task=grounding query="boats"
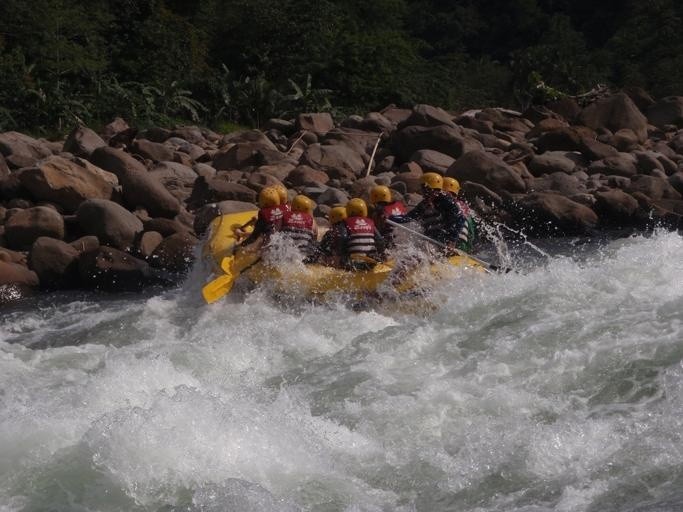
[202,210,487,316]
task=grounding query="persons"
[232,172,476,273]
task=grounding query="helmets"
[258,184,288,208]
[419,172,443,191]
[291,195,312,215]
[346,197,368,217]
[442,177,460,197]
[369,185,392,206]
[329,206,346,225]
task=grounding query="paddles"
[221,235,241,278]
[202,258,262,303]
[384,218,518,275]
